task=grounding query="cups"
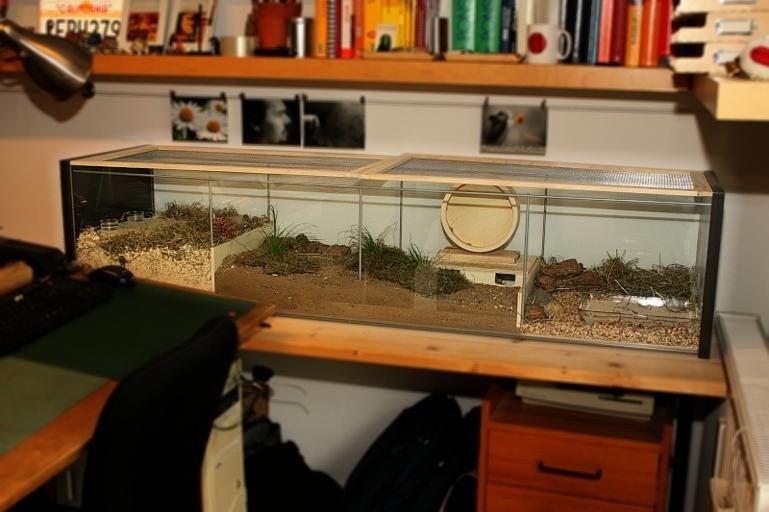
[526,23,571,65]
[288,18,316,57]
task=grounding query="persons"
[169,12,195,49]
[247,99,293,145]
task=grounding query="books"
[313,0,675,70]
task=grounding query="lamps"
[0,19,91,102]
